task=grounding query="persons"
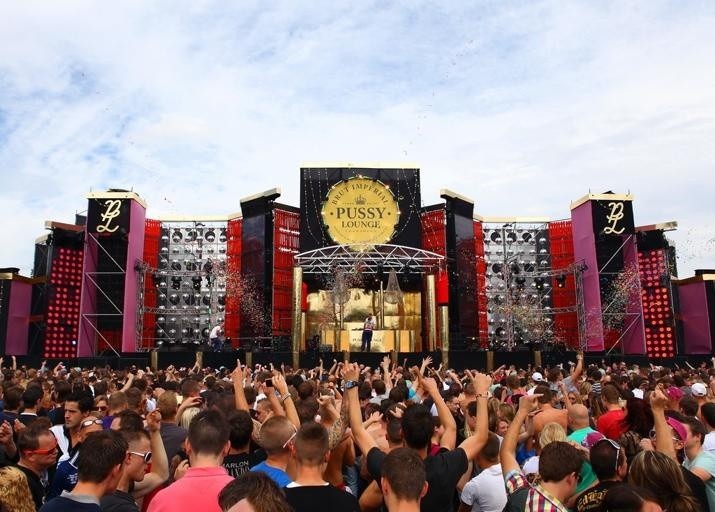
[0,466,34,512]
[126,388,142,415]
[16,427,57,512]
[282,421,362,511]
[443,395,460,422]
[561,360,612,401]
[147,410,237,512]
[629,450,701,512]
[458,435,508,512]
[155,390,189,468]
[500,394,582,512]
[219,471,294,512]
[203,360,345,421]
[96,428,150,511]
[531,386,569,449]
[152,364,204,402]
[492,355,548,403]
[549,355,582,408]
[523,422,567,474]
[361,313,374,353]
[38,429,126,512]
[96,365,152,393]
[17,386,43,428]
[0,363,52,395]
[575,438,627,512]
[180,379,200,428]
[381,448,428,512]
[356,355,475,455]
[621,397,655,464]
[495,417,509,437]
[58,415,103,465]
[0,385,23,423]
[679,396,699,420]
[614,360,715,405]
[48,393,94,461]
[50,364,71,425]
[341,363,492,511]
[93,393,108,417]
[677,417,715,512]
[566,404,607,492]
[103,392,129,428]
[110,410,143,430]
[67,365,96,394]
[597,384,626,440]
[250,415,297,488]
[598,484,662,512]
[700,402,715,455]
[465,401,504,475]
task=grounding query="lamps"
[375,260,385,282]
[403,263,410,284]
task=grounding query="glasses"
[81,419,104,430]
[599,438,621,470]
[283,424,297,449]
[25,443,59,456]
[93,406,109,411]
[649,430,680,442]
[569,397,576,400]
[127,451,153,464]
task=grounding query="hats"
[692,383,707,397]
[532,372,542,381]
[665,417,688,441]
